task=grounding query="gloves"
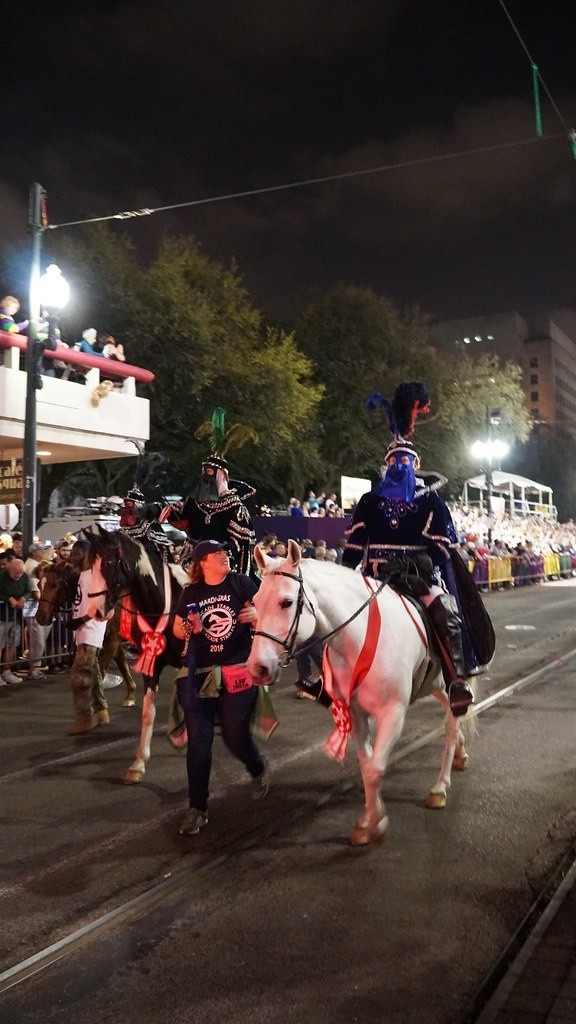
[381,553,413,577]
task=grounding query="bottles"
[434,565,442,586]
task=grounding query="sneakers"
[249,754,270,802]
[0,670,23,686]
[180,807,210,834]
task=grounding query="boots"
[426,594,473,717]
[295,677,332,710]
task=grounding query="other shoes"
[101,672,123,688]
[95,708,110,724]
[44,662,70,674]
[27,669,48,679]
[65,713,98,734]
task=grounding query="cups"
[468,558,475,572]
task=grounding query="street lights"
[475,404,505,551]
[19,184,69,566]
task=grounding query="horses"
[35,523,188,785]
[244,539,478,849]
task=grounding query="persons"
[115,439,474,834]
[0,295,127,393]
[449,507,576,592]
[0,530,110,734]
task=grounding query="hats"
[0,533,11,545]
[194,407,258,475]
[305,538,312,544]
[56,540,69,548]
[124,438,171,504]
[366,380,431,470]
[28,543,43,552]
[191,539,228,562]
[494,539,502,545]
